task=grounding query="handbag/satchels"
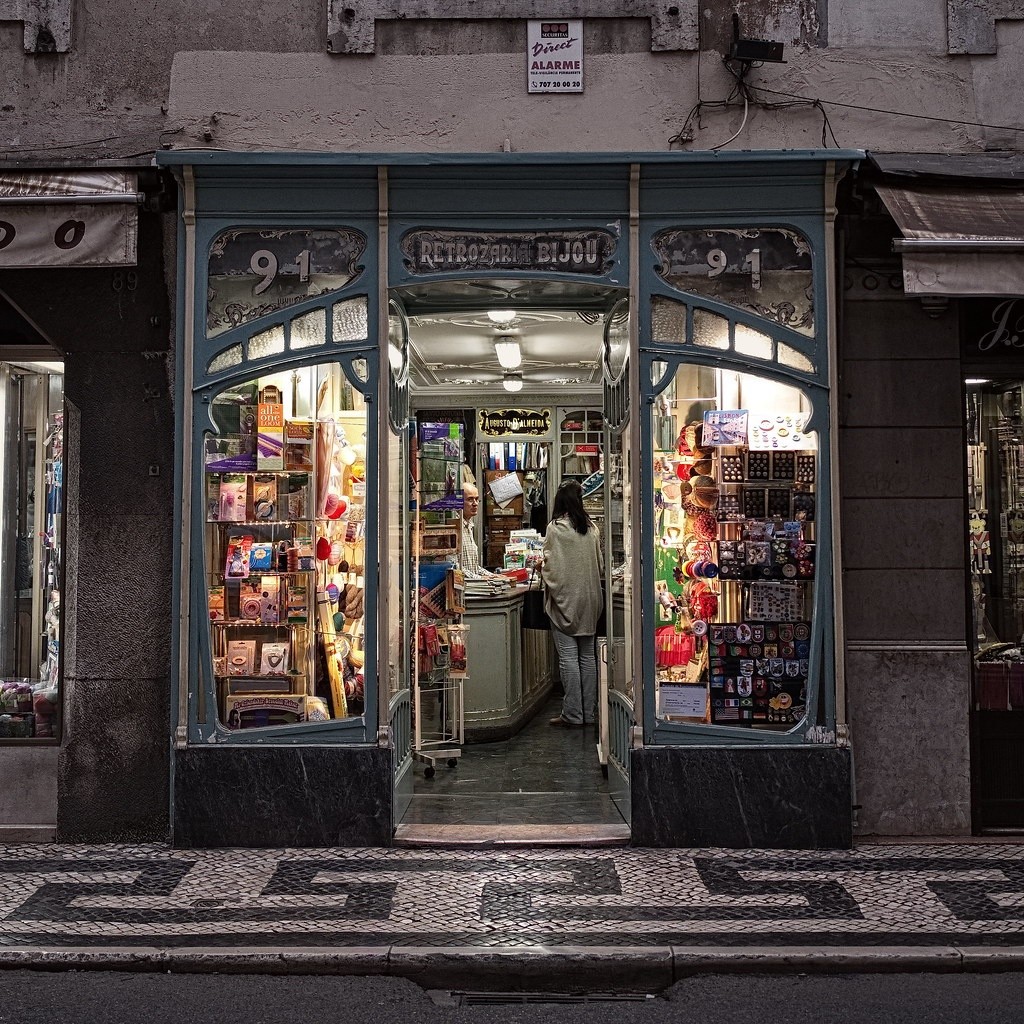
[521,567,557,629]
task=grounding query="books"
[464,577,511,597]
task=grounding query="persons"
[534,478,603,725]
[461,481,507,581]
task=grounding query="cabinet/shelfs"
[458,578,562,730]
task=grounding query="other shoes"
[549,712,578,739]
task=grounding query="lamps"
[502,372,525,392]
[492,335,523,369]
[488,306,519,323]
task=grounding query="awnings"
[869,152,1023,296]
